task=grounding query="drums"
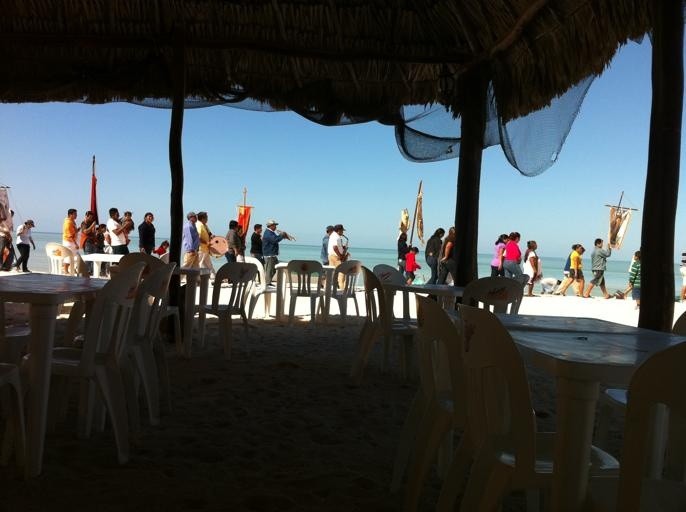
[208,236,229,257]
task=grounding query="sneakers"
[23,270,30,272]
[266,284,275,288]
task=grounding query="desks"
[384,281,463,313]
[445,308,686,512]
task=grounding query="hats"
[187,212,198,219]
[327,226,333,231]
[267,220,278,226]
[28,219,34,227]
[334,225,346,230]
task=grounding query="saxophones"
[342,233,351,266]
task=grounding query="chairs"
[372,263,411,379]
[390,295,473,511]
[461,276,523,315]
[435,303,619,512]
[353,265,418,383]
[589,341,686,512]
[670,312,686,336]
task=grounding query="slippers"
[561,291,565,296]
[584,295,591,298]
[606,295,614,300]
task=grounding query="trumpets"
[275,225,293,241]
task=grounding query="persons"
[680,253,686,302]
[225,221,295,288]
[629,251,642,309]
[320,226,335,290]
[196,212,219,286]
[404,247,421,286]
[491,231,543,296]
[0,210,14,272]
[424,228,444,286]
[328,224,345,291]
[398,233,407,275]
[540,277,561,296]
[13,220,35,273]
[181,212,200,285]
[615,247,642,299]
[431,226,456,302]
[155,240,169,255]
[139,212,156,256]
[586,239,612,297]
[62,208,134,274]
